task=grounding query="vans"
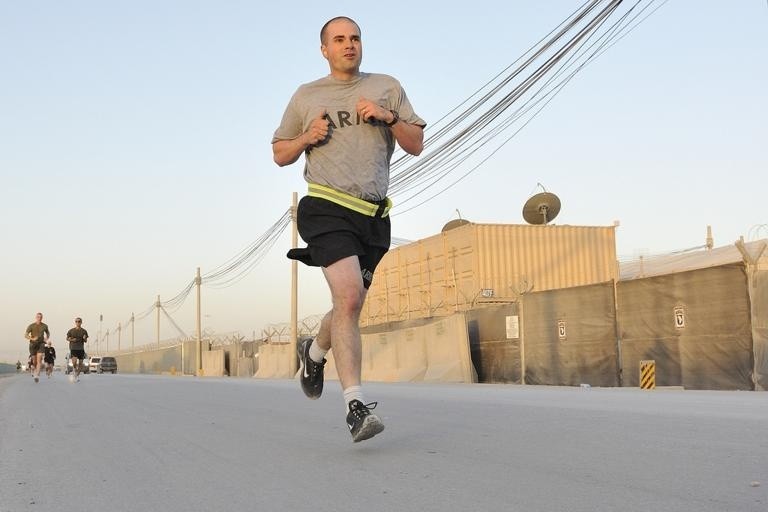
[64,351,91,374]
[88,356,102,373]
[96,356,119,375]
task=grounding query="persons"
[271,16,427,442]
[16,312,88,384]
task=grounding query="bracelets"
[384,109,399,127]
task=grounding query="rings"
[362,109,365,113]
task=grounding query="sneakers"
[346,398,385,443]
[295,336,327,400]
[28,370,81,384]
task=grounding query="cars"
[54,364,62,371]
